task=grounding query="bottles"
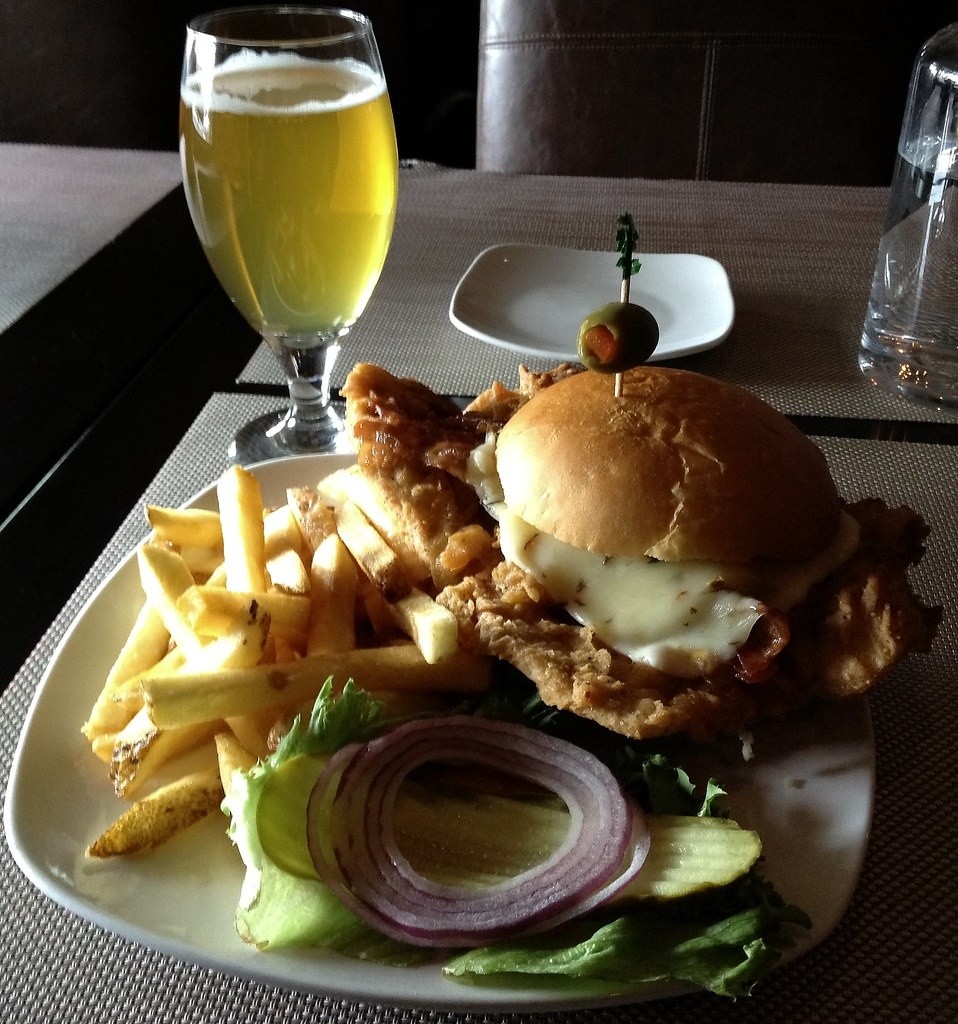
[858,21,958,406]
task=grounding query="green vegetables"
[247,675,812,1001]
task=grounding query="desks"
[1,141,958,1024]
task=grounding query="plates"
[6,453,878,1008]
[447,241,735,366]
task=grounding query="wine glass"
[180,7,399,474]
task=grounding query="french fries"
[86,465,464,861]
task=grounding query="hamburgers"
[484,371,839,682]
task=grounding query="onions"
[303,715,654,945]
